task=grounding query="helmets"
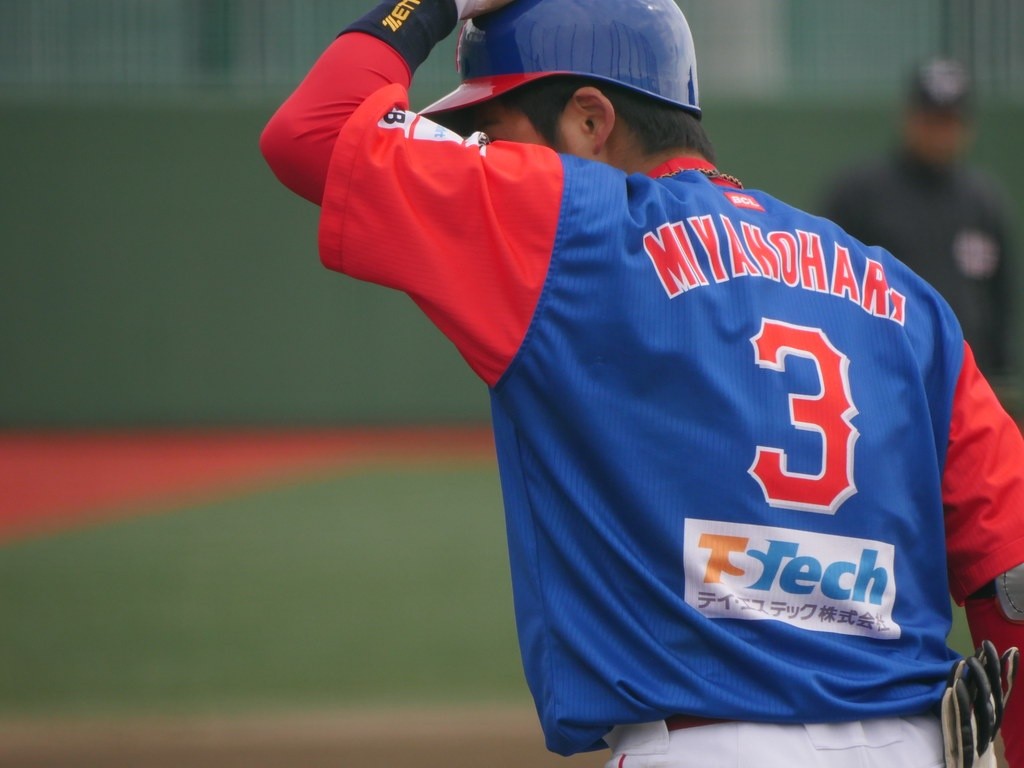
[413,1,705,115]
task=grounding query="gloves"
[941,638,1021,768]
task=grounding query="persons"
[253,0,1024,767]
[814,53,1018,424]
